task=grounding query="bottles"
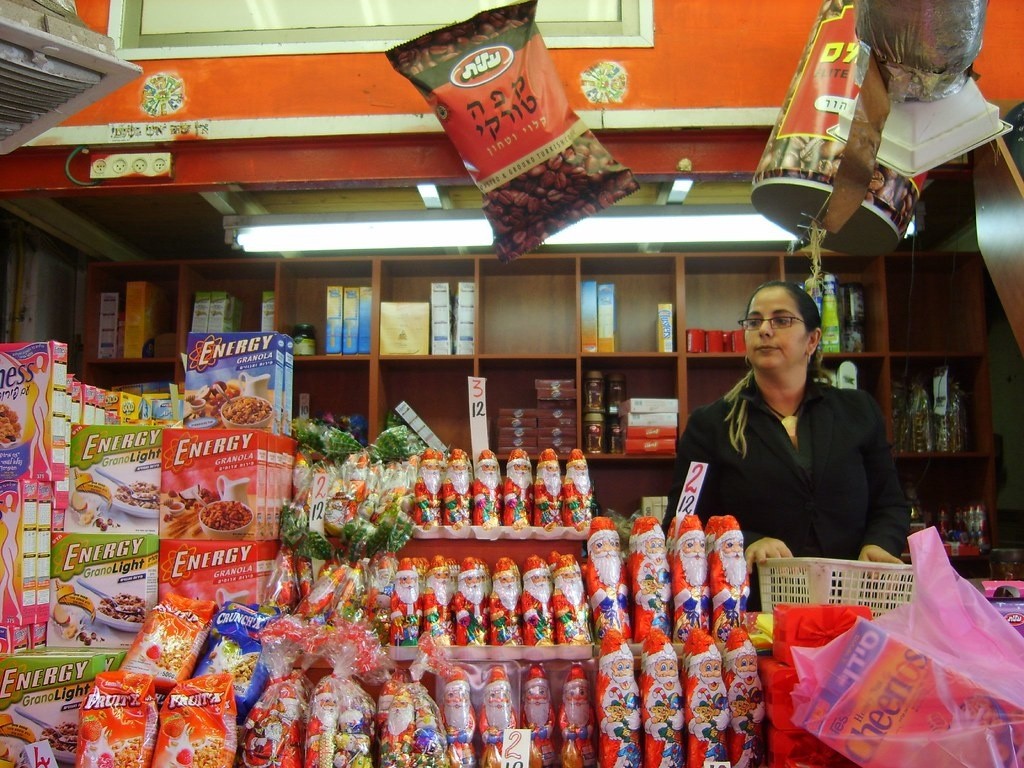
[805,274,841,353]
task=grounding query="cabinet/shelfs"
[82,253,999,581]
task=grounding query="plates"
[24,726,76,763]
[86,594,144,632]
[184,401,191,418]
[101,481,160,518]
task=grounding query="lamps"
[223,204,811,252]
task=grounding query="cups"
[189,399,206,413]
[166,502,185,516]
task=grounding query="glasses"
[737,317,809,331]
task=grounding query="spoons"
[94,468,157,500]
[77,578,141,613]
[14,707,78,744]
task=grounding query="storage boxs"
[655,303,674,352]
[191,291,246,333]
[393,400,448,453]
[300,393,311,420]
[1,341,184,768]
[580,280,619,352]
[494,378,578,455]
[641,496,668,522]
[326,286,372,355]
[98,281,171,359]
[261,291,275,332]
[813,361,857,390]
[159,331,299,621]
[619,398,680,454]
[430,282,475,356]
[380,302,429,355]
[686,329,747,354]
[805,617,1024,768]
[944,543,980,556]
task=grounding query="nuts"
[478,131,638,261]
[752,136,916,239]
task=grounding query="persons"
[660,281,909,575]
[243,447,765,768]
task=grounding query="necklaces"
[763,397,804,437]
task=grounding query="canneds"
[579,368,626,455]
[687,328,746,353]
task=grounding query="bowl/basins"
[198,499,254,540]
[221,395,273,429]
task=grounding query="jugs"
[216,475,250,501]
[238,372,271,400]
[215,588,249,610]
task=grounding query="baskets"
[755,557,915,620]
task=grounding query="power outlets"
[89,153,174,180]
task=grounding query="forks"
[186,395,195,402]
[159,500,173,506]
